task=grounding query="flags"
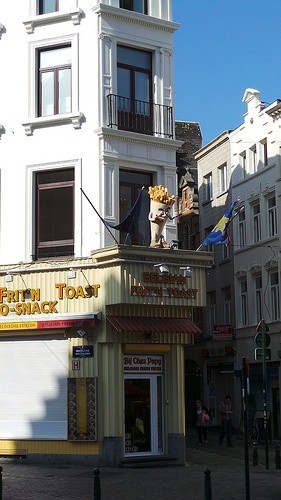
[202,201,238,247]
[104,191,142,232]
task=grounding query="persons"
[192,400,211,446]
[219,394,234,448]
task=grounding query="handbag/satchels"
[203,413,210,422]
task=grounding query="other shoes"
[219,438,222,445]
[227,444,233,448]
